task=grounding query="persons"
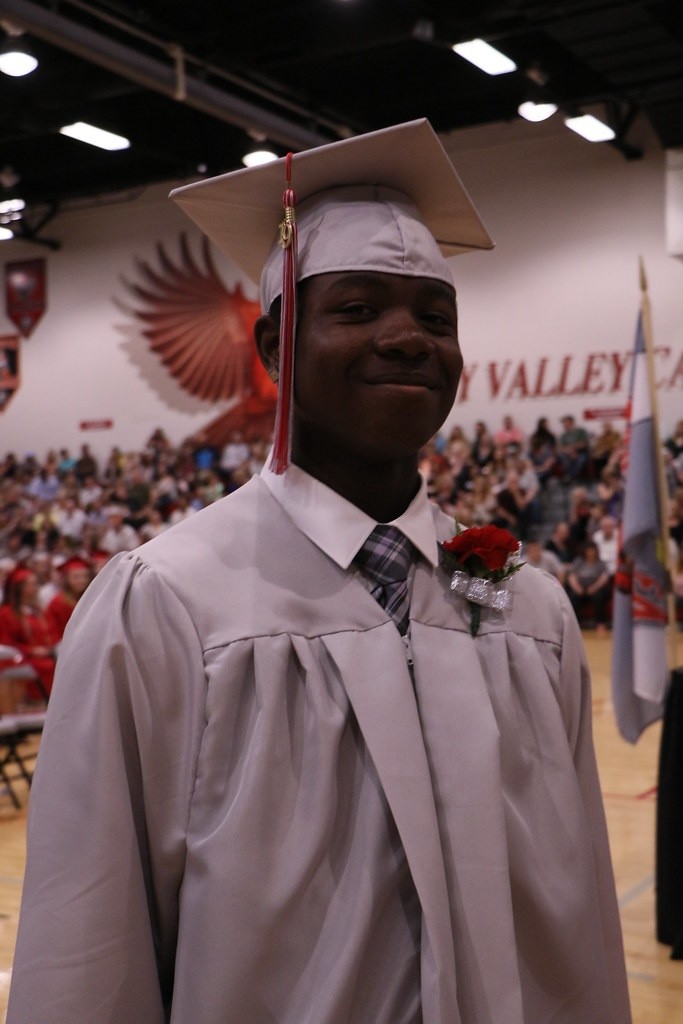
[0,115,650,1024]
[649,533,683,970]
[0,398,683,711]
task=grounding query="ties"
[358,526,416,638]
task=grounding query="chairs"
[0,646,49,809]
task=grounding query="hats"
[4,549,111,584]
[167,118,496,475]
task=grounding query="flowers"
[435,515,529,639]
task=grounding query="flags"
[0,332,22,414]
[605,299,668,748]
[2,255,50,341]
